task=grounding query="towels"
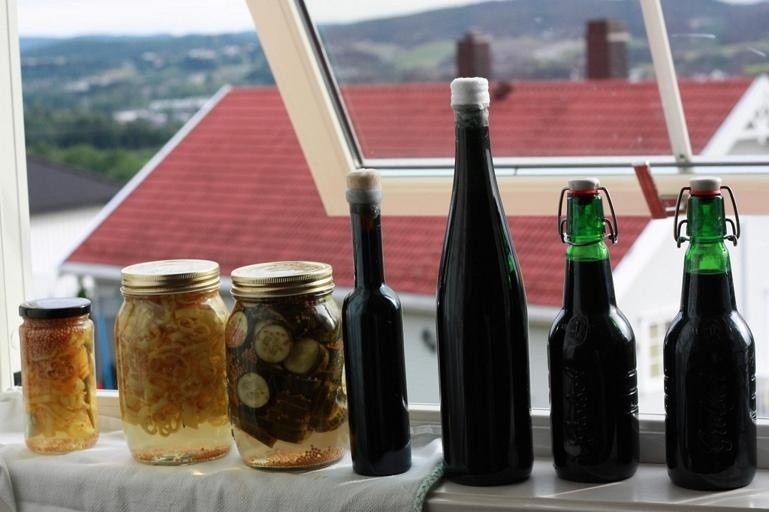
[1,411,445,511]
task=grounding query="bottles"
[543,176,641,485]
[657,176,761,491]
[433,76,537,489]
[336,168,414,478]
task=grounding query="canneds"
[18,295,98,453]
[224,256,347,467]
[113,258,228,465]
